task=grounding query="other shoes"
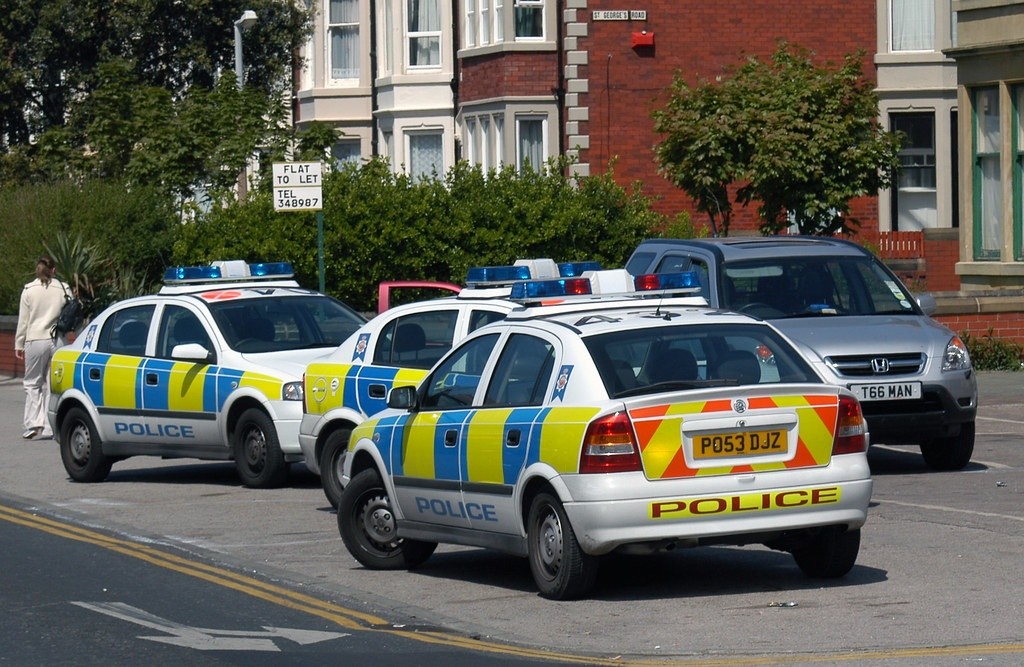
[37,434,53,440]
[23,426,38,439]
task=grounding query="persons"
[14,257,75,438]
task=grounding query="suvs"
[624,235,980,469]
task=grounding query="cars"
[296,257,705,506]
[44,259,392,490]
[337,268,875,601]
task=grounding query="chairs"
[118,321,149,351]
[598,360,637,393]
[237,318,275,341]
[712,350,761,384]
[393,323,426,362]
[173,318,208,346]
[647,348,698,384]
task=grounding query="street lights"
[233,11,258,206]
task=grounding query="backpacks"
[50,282,84,339]
